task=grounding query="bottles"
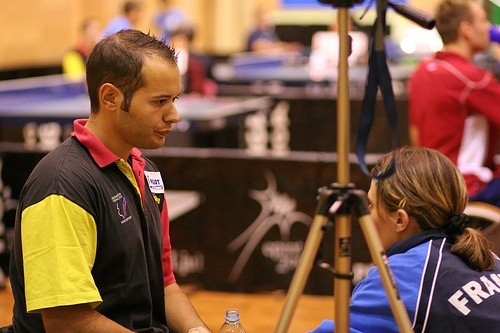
[218,310,246,333]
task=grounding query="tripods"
[274,0,416,333]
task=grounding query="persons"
[149,1,188,49]
[98,0,143,43]
[307,147,500,333]
[61,17,102,79]
[10,29,213,333]
[407,0,500,239]
